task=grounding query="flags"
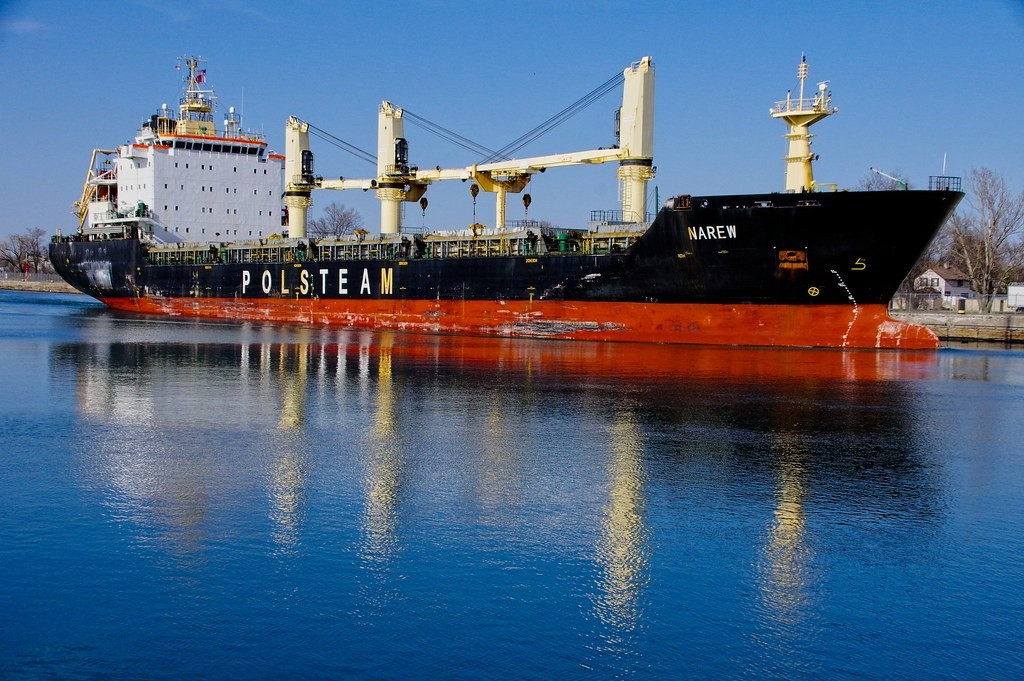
[196,69,206,75]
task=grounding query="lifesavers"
[290,253,295,260]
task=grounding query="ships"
[43,57,967,347]
